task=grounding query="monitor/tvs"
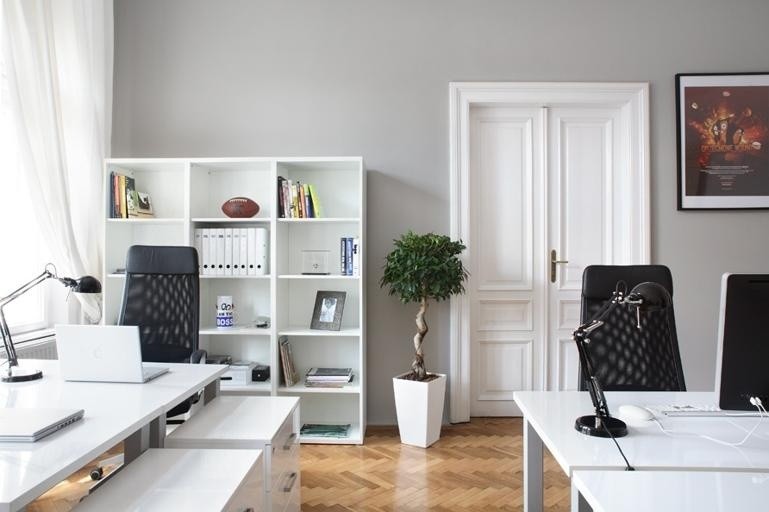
[713,272,769,411]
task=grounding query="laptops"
[0,407,84,443]
[53,323,171,384]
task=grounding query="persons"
[320,298,336,322]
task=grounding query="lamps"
[0,263,102,381]
[573,280,672,437]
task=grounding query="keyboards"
[655,399,769,418]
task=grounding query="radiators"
[0,334,58,360]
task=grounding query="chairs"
[577,264,687,391]
[80,245,232,501]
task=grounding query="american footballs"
[221,198,259,218]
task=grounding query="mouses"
[619,404,654,422]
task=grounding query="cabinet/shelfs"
[68,448,266,512]
[103,157,368,445]
[164,395,302,511]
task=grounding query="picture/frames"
[310,291,345,331]
[675,72,769,211]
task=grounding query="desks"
[0,405,164,511]
[572,469,769,512]
[512,391,769,512]
[0,357,230,448]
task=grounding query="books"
[277,175,320,218]
[277,335,356,388]
[340,236,360,277]
[110,171,154,219]
[300,423,352,439]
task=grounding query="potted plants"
[378,226,472,451]
